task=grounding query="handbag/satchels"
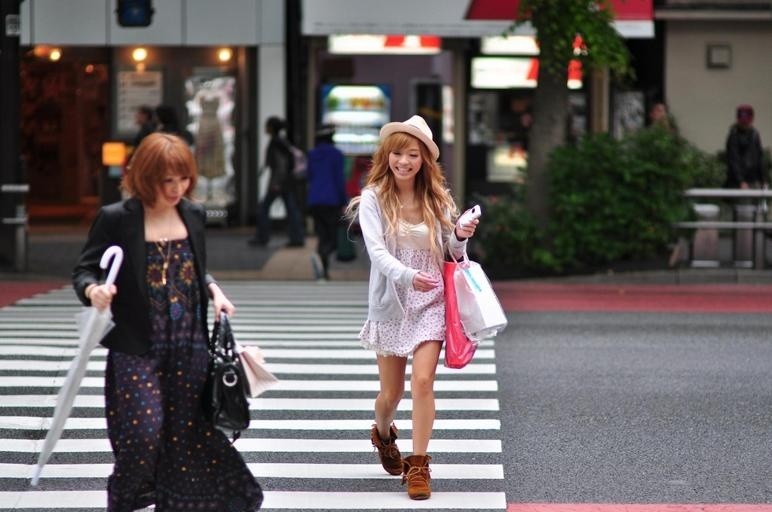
[208,311,250,431]
[336,224,357,263]
[454,261,509,345]
[237,344,278,399]
[443,261,478,368]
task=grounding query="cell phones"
[459,204,482,229]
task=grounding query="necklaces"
[149,207,173,286]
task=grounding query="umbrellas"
[29,245,124,487]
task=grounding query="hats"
[380,115,440,161]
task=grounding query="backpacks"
[285,142,310,184]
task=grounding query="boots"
[371,423,403,476]
[400,455,432,499]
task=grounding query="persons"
[247,92,441,281]
[647,102,671,133]
[721,104,765,270]
[509,95,533,148]
[115,106,157,155]
[154,105,197,147]
[70,132,264,512]
[343,113,479,500]
[197,76,225,177]
